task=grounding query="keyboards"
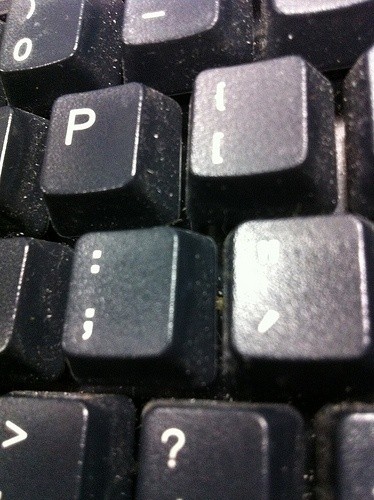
[1,1,374,500]
